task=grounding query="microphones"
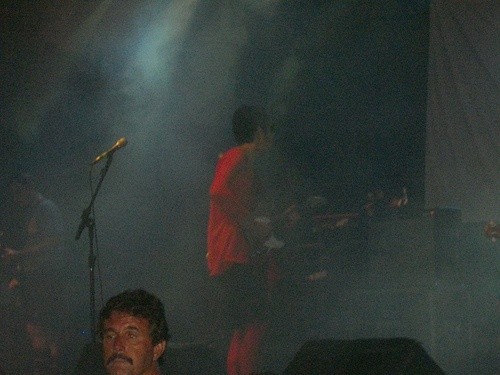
[88,137,127,165]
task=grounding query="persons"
[207,103,301,374]
[1,171,68,375]
[98,288,170,375]
